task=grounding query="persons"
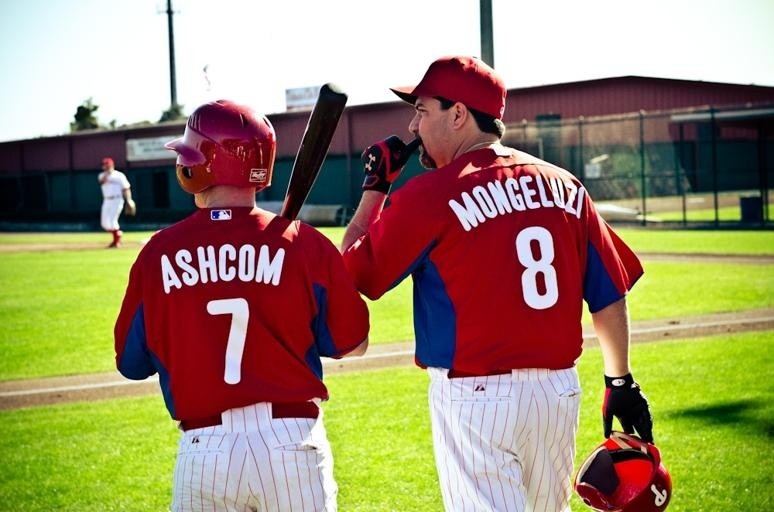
[114,100,371,512]
[97,157,135,247]
[340,55,654,512]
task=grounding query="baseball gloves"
[125,200,136,217]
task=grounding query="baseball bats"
[280,84,348,220]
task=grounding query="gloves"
[602,375,652,444]
[360,136,421,196]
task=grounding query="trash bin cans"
[740,196,762,223]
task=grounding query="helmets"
[575,430,671,512]
[165,99,277,194]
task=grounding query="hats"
[390,56,508,120]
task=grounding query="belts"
[182,402,319,432]
